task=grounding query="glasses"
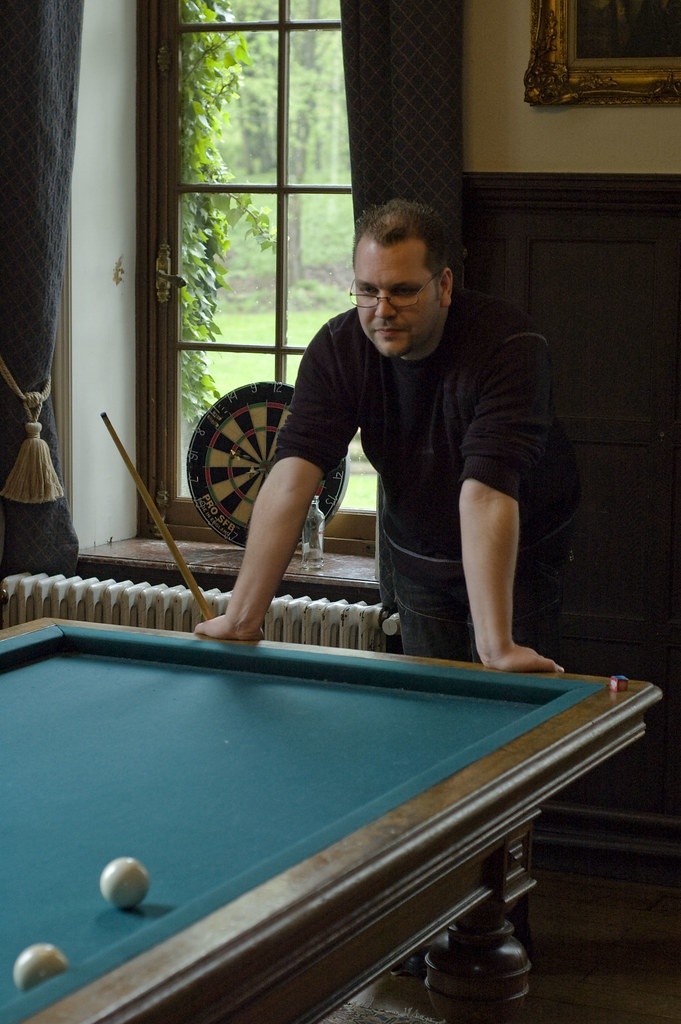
[350,272,439,308]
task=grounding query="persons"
[194,201,580,980]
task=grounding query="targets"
[186,381,352,549]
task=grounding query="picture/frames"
[523,0,681,107]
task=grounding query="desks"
[0,616,662,1024]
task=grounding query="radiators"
[0,572,402,651]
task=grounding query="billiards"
[99,856,150,912]
[12,941,68,992]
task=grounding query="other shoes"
[406,952,427,980]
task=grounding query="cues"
[100,412,215,621]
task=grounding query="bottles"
[301,496,324,569]
